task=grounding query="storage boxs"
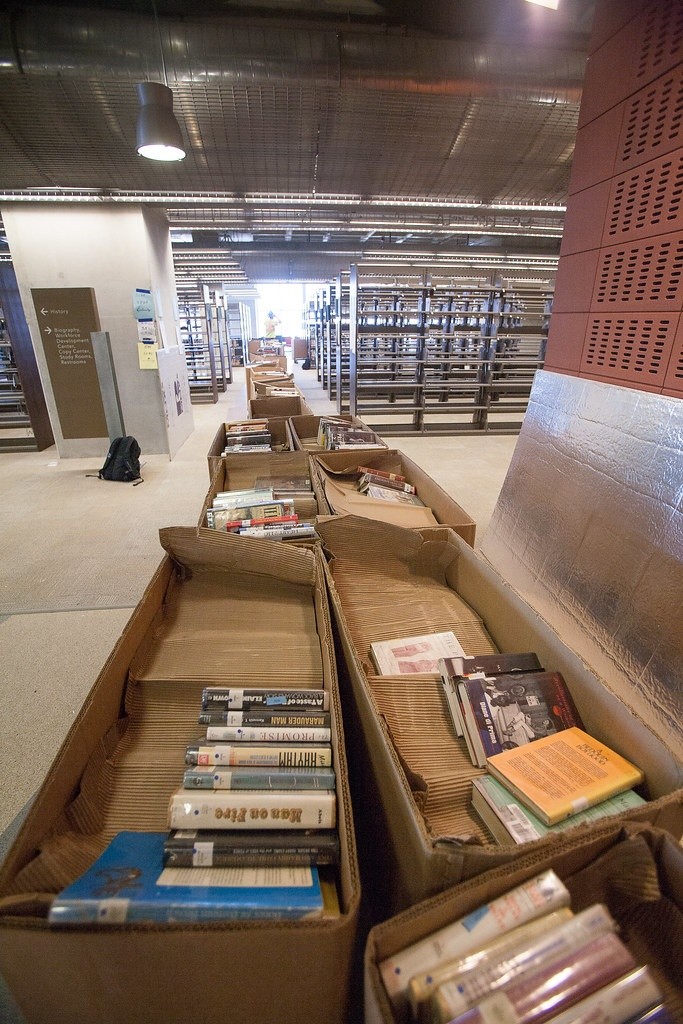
[1,353,683,1024]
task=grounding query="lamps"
[136,81,186,162]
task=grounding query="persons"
[264,310,281,338]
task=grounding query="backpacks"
[99,436,141,482]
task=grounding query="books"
[221,420,271,456]
[317,416,386,450]
[380,869,672,1024]
[471,726,648,847]
[370,631,466,676]
[438,652,587,767]
[50,686,337,922]
[261,371,285,375]
[208,475,317,541]
[271,388,298,397]
[347,466,425,507]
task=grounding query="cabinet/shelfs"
[173,260,559,436]
